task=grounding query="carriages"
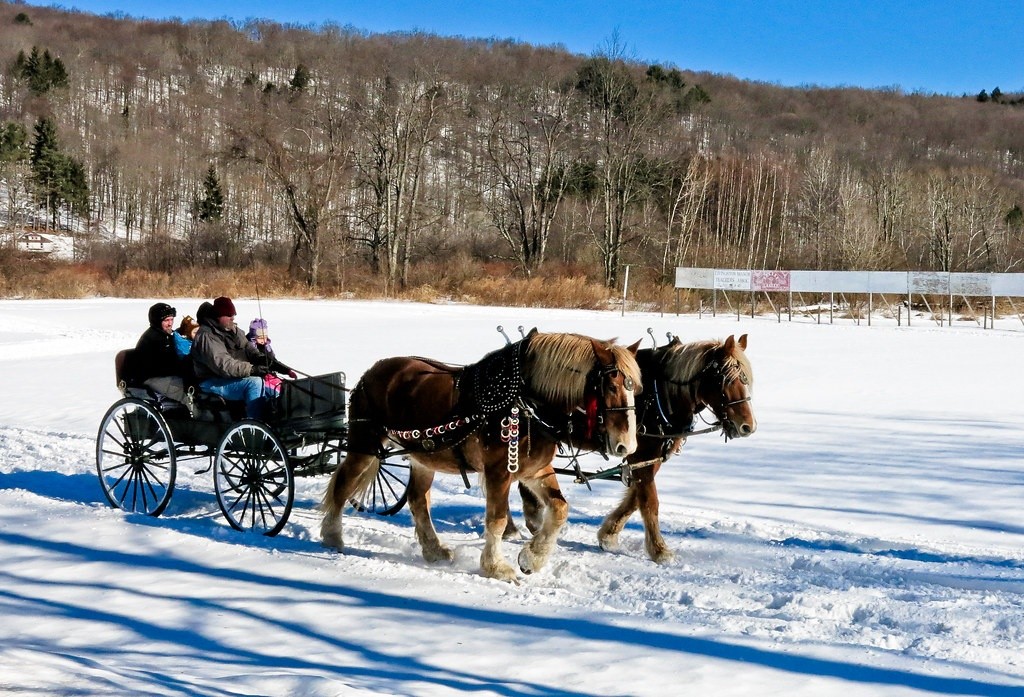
[95,325,758,582]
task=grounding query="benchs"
[186,383,286,412]
[114,350,185,411]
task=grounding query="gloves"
[286,369,298,380]
[254,365,269,377]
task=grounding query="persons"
[134,303,202,419]
[245,318,282,392]
[191,297,297,420]
[179,316,200,341]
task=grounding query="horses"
[519,332,756,569]
[319,330,644,583]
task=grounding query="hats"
[180,315,200,342]
[149,303,176,331]
[214,298,237,318]
[249,319,272,354]
